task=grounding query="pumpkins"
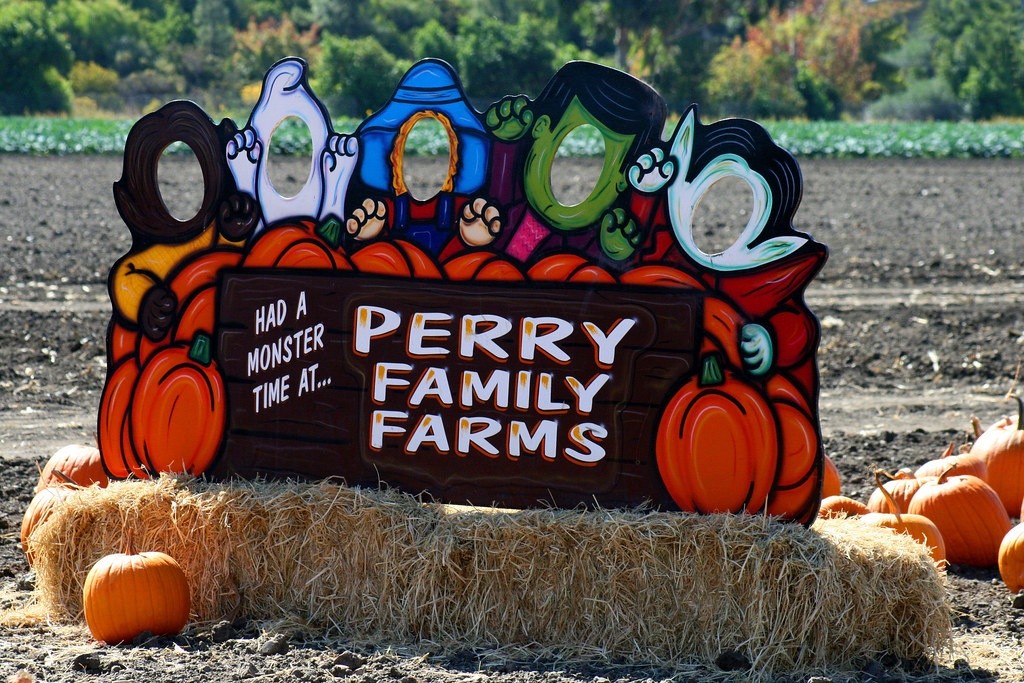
[20,431,108,565]
[83,526,190,644]
[816,394,1024,594]
